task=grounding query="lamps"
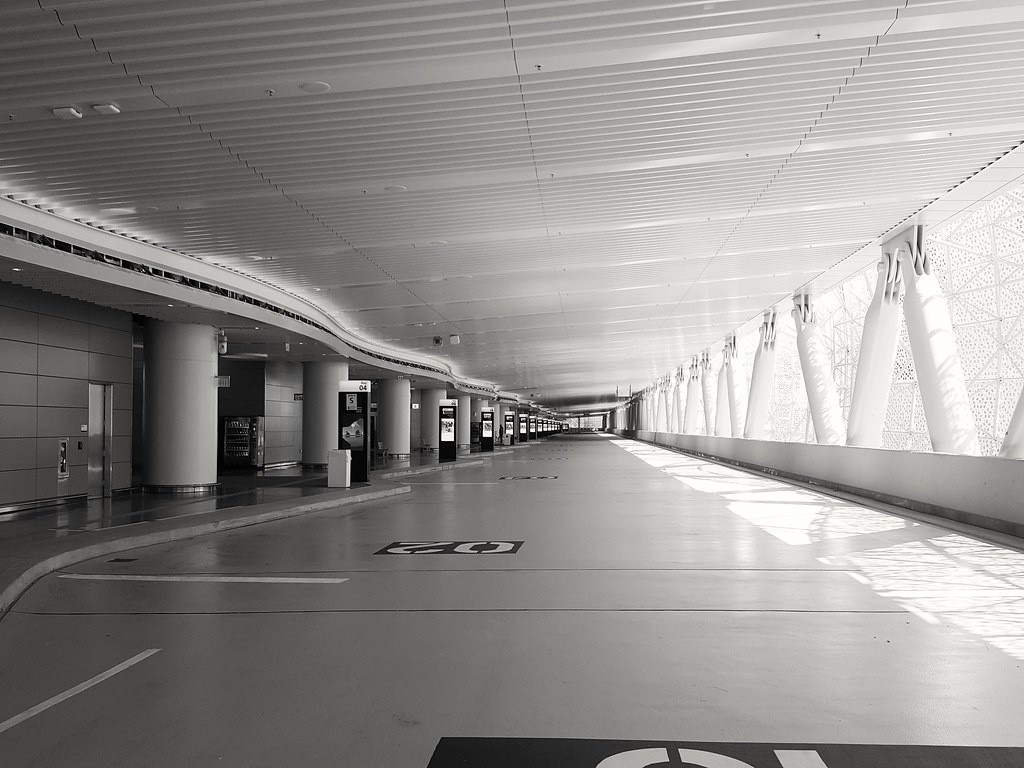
[215,330,228,354]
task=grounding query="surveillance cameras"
[433,337,443,346]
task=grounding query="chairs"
[376,440,391,458]
[421,439,432,452]
